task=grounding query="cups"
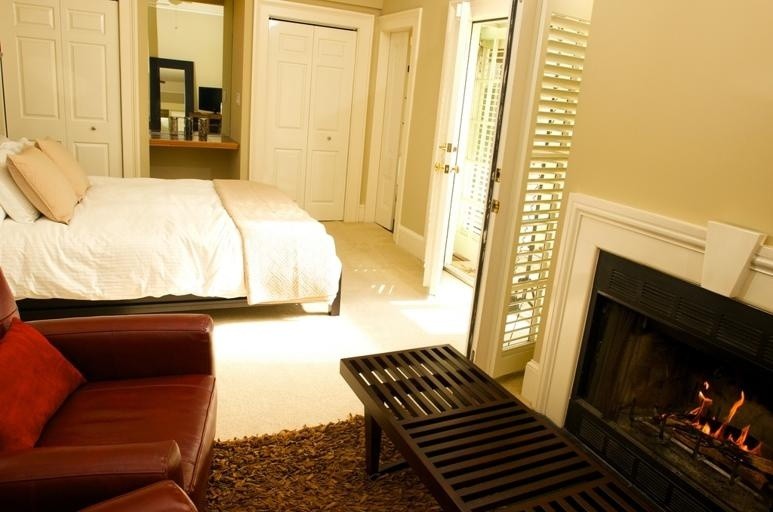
[184,117,193,141]
[168,117,177,137]
[198,117,208,141]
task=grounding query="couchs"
[75,479,198,512]
[0,264,218,512]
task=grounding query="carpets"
[210,403,445,511]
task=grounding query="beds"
[0,136,343,317]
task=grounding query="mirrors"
[148,0,224,138]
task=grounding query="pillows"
[7,143,84,226]
[34,139,91,196]
[0,143,42,226]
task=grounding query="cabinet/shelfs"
[267,17,357,222]
[0,0,124,179]
